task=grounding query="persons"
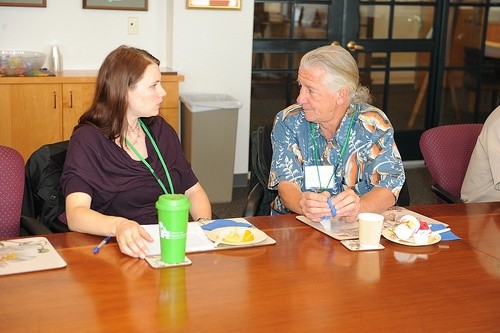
[60,45,212,259]
[268,45,407,223]
[460,101,500,202]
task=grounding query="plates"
[208,226,266,245]
[382,226,441,247]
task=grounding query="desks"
[0,199,500,333]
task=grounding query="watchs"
[197,217,210,221]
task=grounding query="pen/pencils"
[327,197,336,216]
[93,237,110,253]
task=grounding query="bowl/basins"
[0,50,46,76]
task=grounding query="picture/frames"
[187,0,241,10]
[82,0,149,12]
[0,0,47,7]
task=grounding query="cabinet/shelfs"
[0,70,185,165]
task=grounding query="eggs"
[394,214,420,240]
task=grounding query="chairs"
[0,123,485,240]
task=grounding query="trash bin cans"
[180,93,240,204]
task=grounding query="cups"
[155,193,191,264]
[394,215,421,241]
[49,45,63,73]
[358,213,384,249]
